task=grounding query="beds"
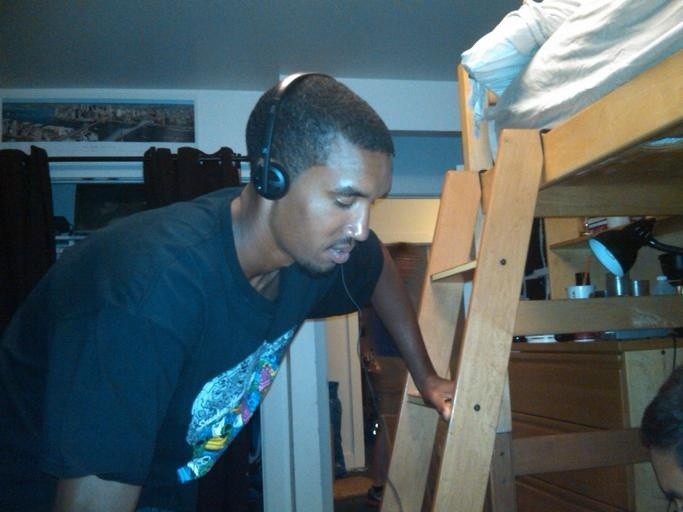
[458,1,683,213]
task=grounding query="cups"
[565,284,596,343]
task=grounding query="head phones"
[253,71,333,200]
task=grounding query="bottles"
[648,275,676,337]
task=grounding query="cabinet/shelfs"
[508,336,683,512]
[543,216,683,299]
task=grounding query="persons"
[639,363,683,512]
[0,73,456,512]
